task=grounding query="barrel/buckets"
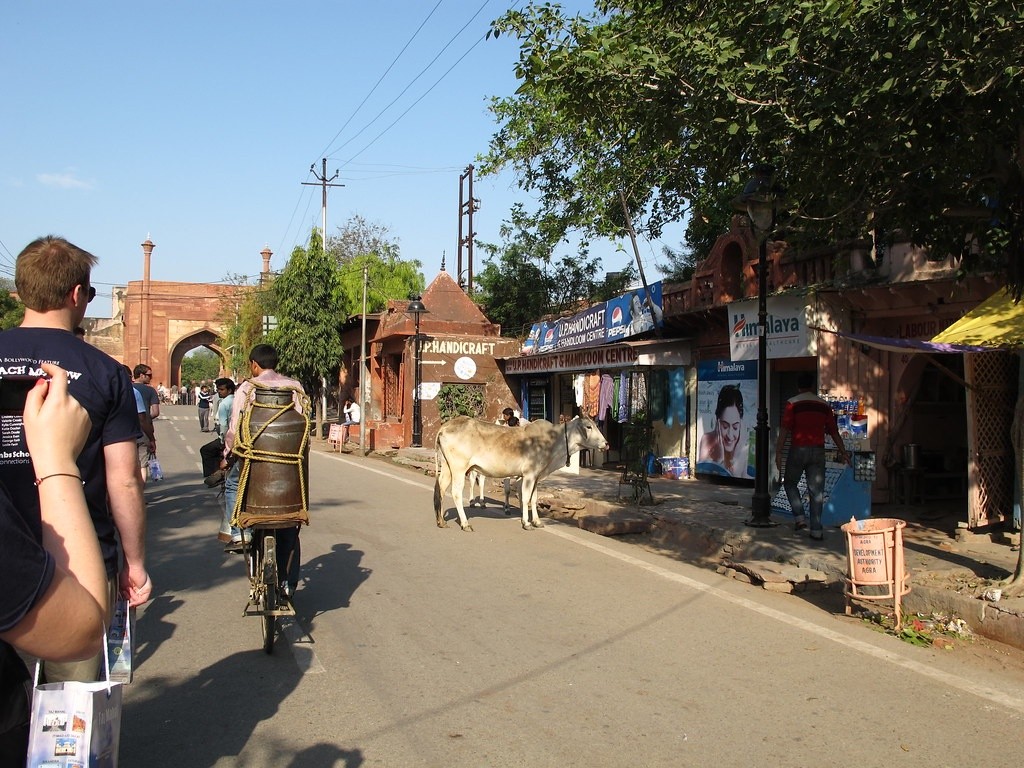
[238,392,309,523]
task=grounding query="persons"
[158,382,165,403]
[342,398,360,440]
[0,363,110,768]
[699,385,749,477]
[776,379,851,539]
[172,379,223,435]
[1,236,160,682]
[624,294,662,337]
[200,378,235,488]
[224,345,306,609]
[503,409,530,426]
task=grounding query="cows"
[434,405,610,532]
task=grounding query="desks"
[893,466,966,506]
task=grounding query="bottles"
[817,393,866,416]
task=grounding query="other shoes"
[204,470,227,489]
[810,534,823,540]
[795,520,808,530]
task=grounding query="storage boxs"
[660,456,689,480]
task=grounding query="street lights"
[402,295,431,448]
[738,163,782,528]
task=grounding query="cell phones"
[0,375,51,417]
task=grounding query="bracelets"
[34,473,85,488]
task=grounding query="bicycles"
[222,458,298,654]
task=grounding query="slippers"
[224,540,248,552]
[280,595,294,607]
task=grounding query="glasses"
[144,373,152,377]
[84,286,96,302]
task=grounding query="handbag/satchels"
[148,452,163,482]
[26,626,123,768]
[100,591,135,684]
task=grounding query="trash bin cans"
[840,518,914,633]
[328,423,352,454]
